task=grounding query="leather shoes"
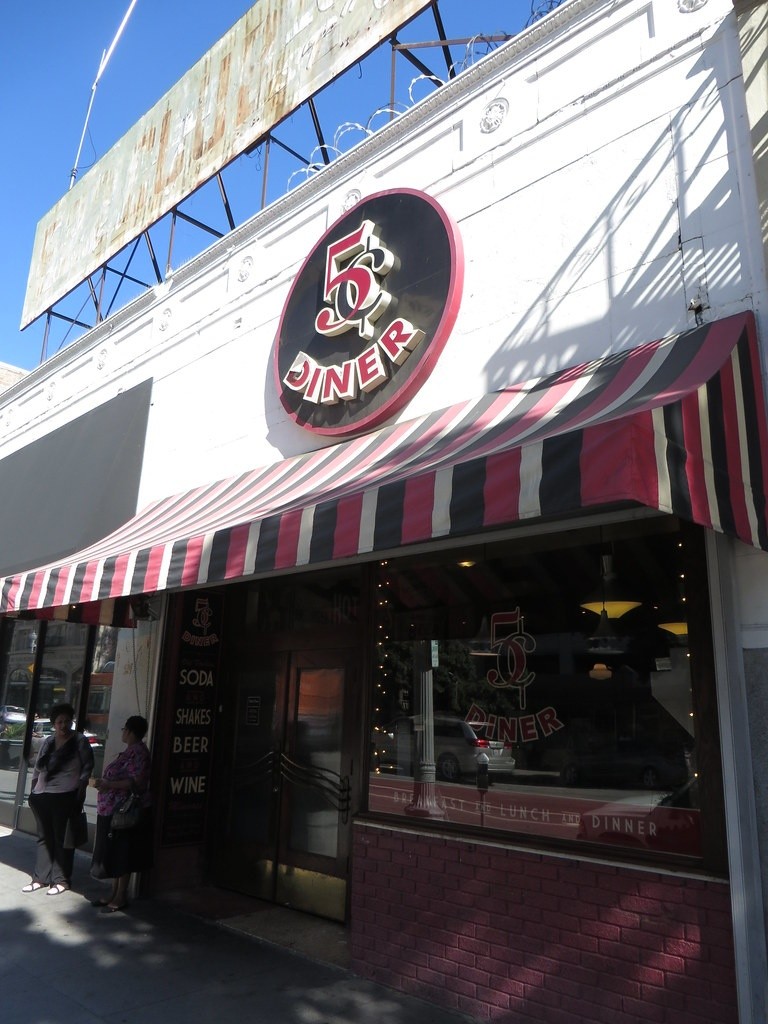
[91,895,118,907]
[101,900,128,913]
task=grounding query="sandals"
[46,884,66,895]
[22,881,49,892]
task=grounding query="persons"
[22,704,94,895]
[92,715,150,914]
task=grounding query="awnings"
[0,311,767,626]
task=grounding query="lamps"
[454,523,689,677]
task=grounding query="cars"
[0,704,26,723]
[0,718,103,764]
[371,715,515,783]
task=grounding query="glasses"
[35,716,39,718]
[121,728,127,731]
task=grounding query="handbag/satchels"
[110,777,141,830]
[63,798,89,849]
